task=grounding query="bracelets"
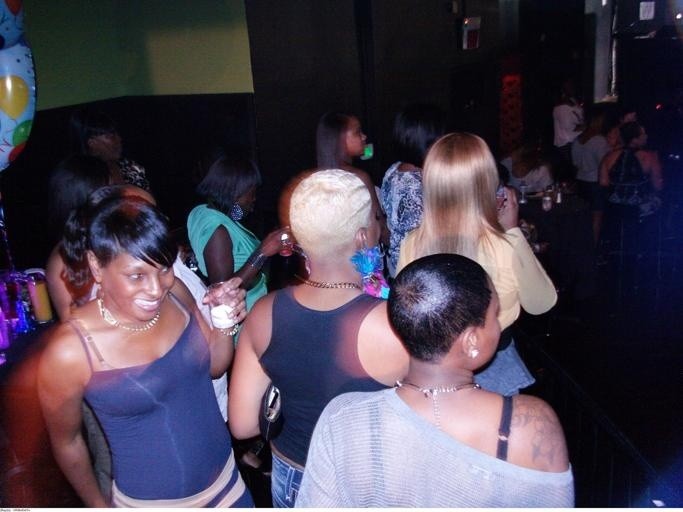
[219,323,240,336]
[247,249,267,272]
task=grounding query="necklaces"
[96,298,161,331]
[302,279,364,291]
[397,377,481,436]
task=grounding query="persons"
[381,104,450,279]
[185,153,297,471]
[1,185,230,507]
[548,75,584,167]
[228,167,410,508]
[599,122,665,219]
[396,128,558,397]
[36,196,254,507]
[316,110,391,249]
[294,254,575,509]
[498,136,554,195]
[52,122,151,244]
[483,133,510,185]
[608,108,638,152]
[571,111,614,246]
[1,131,231,211]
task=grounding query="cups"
[206,282,238,336]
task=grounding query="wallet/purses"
[259,383,282,441]
